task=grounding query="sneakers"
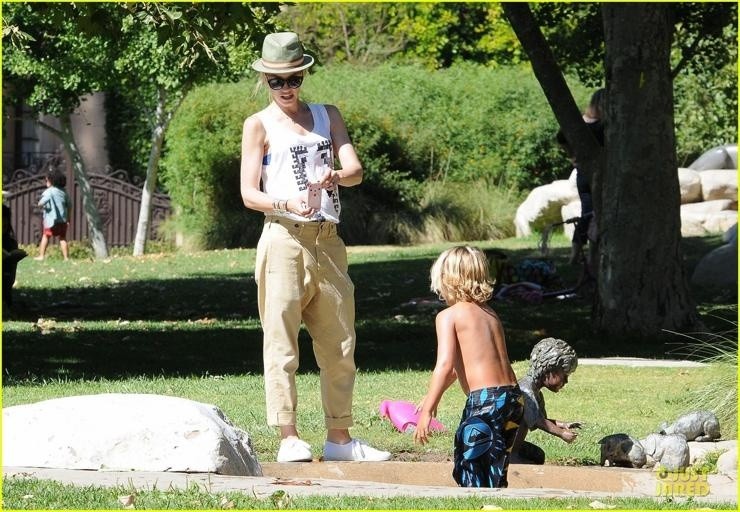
[277,435,313,462]
[323,438,393,462]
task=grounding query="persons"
[567,88,607,264]
[239,33,397,464]
[510,335,583,465]
[412,243,525,484]
[33,169,72,262]
[2,202,28,305]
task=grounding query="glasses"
[265,71,304,90]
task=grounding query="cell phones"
[306,180,322,210]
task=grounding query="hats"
[47,169,66,188]
[251,31,315,75]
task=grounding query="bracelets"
[272,199,289,212]
